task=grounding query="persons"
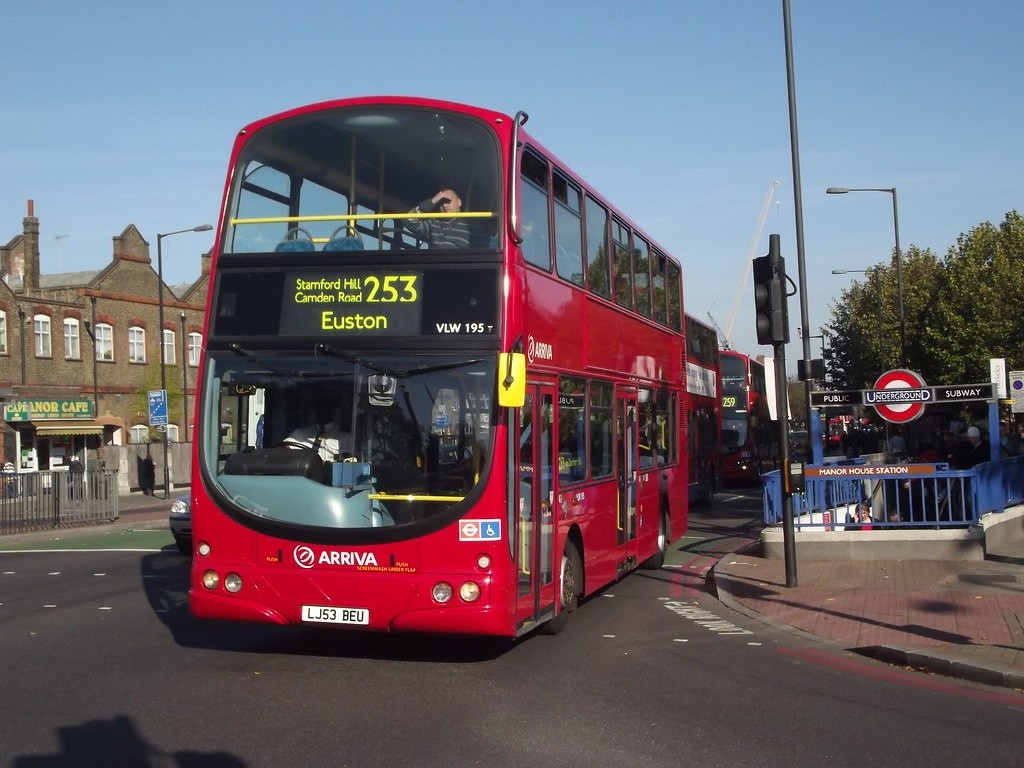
[613,258,630,305]
[275,397,356,463]
[403,188,491,249]
[516,215,546,269]
[828,419,1024,508]
[846,502,881,530]
[69,456,84,502]
[453,421,486,464]
[144,453,157,495]
[889,510,912,529]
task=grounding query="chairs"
[275,224,670,526]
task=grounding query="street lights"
[832,269,892,450]
[156,224,214,499]
[828,185,908,371]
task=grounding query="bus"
[187,94,692,649]
[718,350,783,491]
[681,312,723,509]
